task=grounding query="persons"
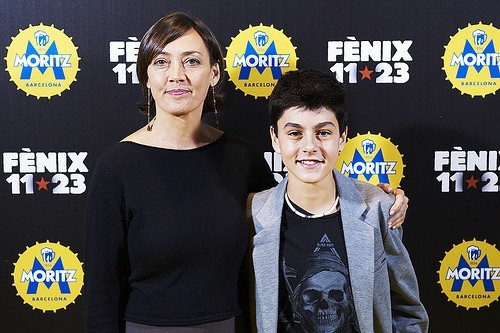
[246,67,428,333]
[84,11,407,332]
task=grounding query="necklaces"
[285,188,340,218]
